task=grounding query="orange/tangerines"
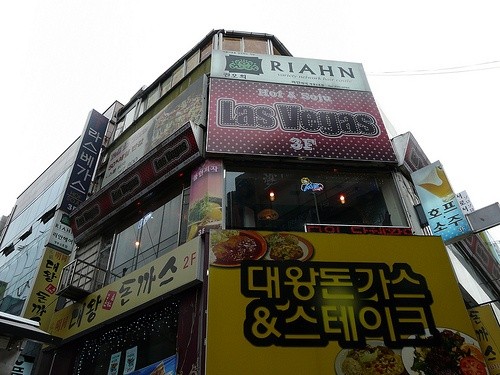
[460,344,487,365]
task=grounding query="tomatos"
[460,357,487,375]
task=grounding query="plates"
[334,338,407,375]
[210,230,267,268]
[402,327,483,375]
[263,233,315,262]
